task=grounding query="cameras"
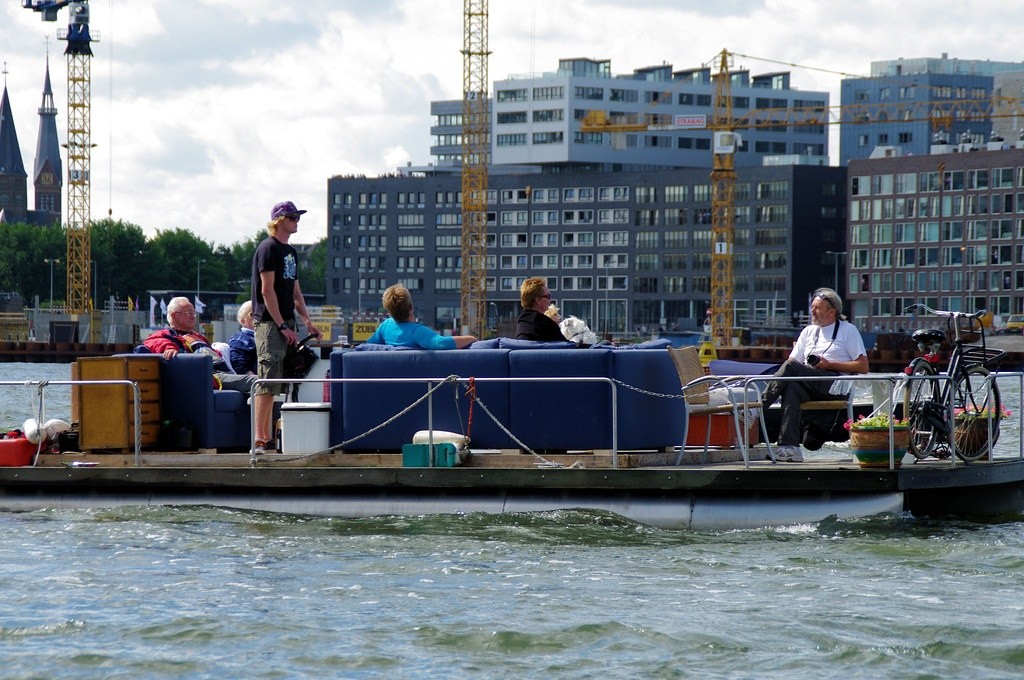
[807,355,820,366]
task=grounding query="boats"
[708,358,938,445]
[0,339,1024,534]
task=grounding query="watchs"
[280,323,288,330]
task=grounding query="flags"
[127,296,207,329]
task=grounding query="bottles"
[412,430,470,444]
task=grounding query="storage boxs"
[58,430,79,454]
[282,403,330,454]
[686,387,758,448]
[0,437,46,467]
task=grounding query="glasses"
[245,313,252,322]
[175,310,196,317]
[817,292,836,310]
[541,292,551,301]
[285,215,300,222]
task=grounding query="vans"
[1006,313,1024,333]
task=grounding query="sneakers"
[249,438,266,456]
[765,444,803,462]
[727,401,756,430]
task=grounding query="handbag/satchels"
[282,332,321,378]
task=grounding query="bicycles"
[903,302,1002,466]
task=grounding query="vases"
[849,429,909,467]
[954,418,1000,456]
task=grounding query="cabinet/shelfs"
[77,354,160,454]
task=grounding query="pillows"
[463,337,576,349]
[589,338,672,349]
[355,343,411,350]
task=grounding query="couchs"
[330,349,686,451]
[135,345,253,455]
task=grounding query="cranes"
[22,0,101,315]
[580,46,1024,347]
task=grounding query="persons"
[251,201,323,456]
[516,277,575,342]
[728,288,870,462]
[544,304,562,322]
[142,297,319,455]
[367,284,477,350]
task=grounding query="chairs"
[667,345,776,465]
[794,331,878,429]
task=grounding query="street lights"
[197,259,207,325]
[43,258,61,309]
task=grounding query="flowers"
[844,411,910,430]
[944,398,1012,421]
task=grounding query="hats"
[544,304,560,318]
[271,201,308,222]
